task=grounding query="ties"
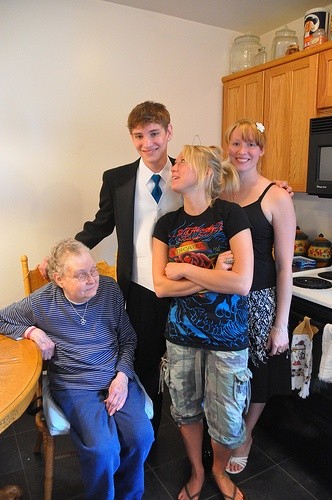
[151,174,162,204]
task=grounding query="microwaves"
[307,116,332,198]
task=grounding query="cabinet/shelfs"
[222,41,332,194]
[280,298,332,490]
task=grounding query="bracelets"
[272,326,288,332]
[25,327,37,340]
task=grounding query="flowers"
[256,123,266,133]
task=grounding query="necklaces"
[232,173,261,199]
[69,302,89,326]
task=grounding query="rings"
[277,350,281,353]
[224,258,234,264]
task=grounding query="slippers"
[225,455,248,473]
[177,474,209,500]
[211,474,247,500]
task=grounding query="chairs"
[20,253,154,500]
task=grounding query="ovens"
[269,309,332,494]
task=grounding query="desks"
[0,330,42,500]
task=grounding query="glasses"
[62,266,99,282]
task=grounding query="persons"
[37,101,294,463]
[0,240,155,500]
[221,119,297,474]
[152,145,254,500]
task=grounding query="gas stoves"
[290,265,332,326]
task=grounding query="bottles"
[269,24,299,60]
[293,225,309,257]
[307,233,332,268]
[309,29,327,47]
[254,47,267,66]
[230,33,263,74]
